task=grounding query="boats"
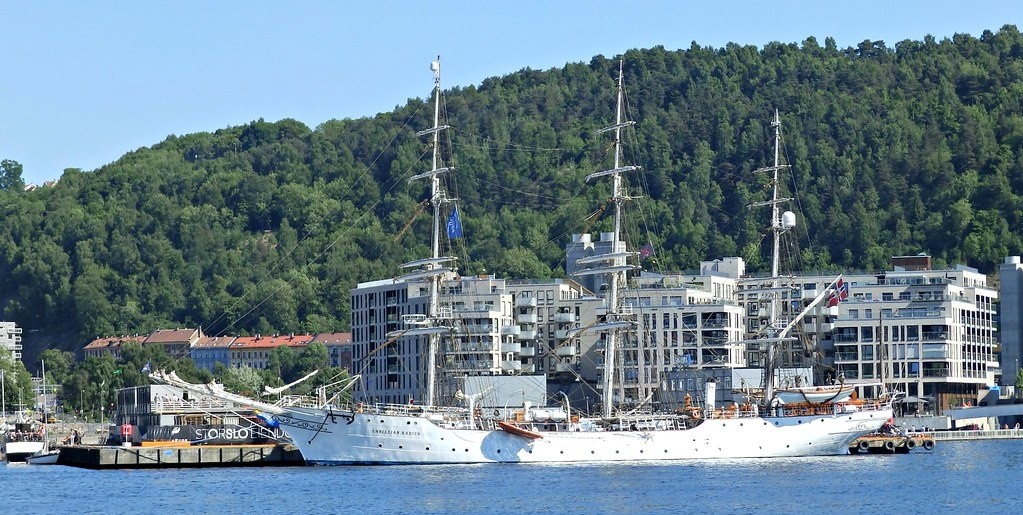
[1,369,46,462]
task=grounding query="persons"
[972,423,983,430]
[11,429,79,445]
[718,400,831,416]
[875,400,880,410]
[357,403,363,413]
[878,418,934,434]
[967,400,972,407]
[1004,424,1009,430]
[153,393,218,404]
[1015,423,1020,429]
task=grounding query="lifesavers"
[691,409,701,419]
[883,416,894,427]
[494,410,500,416]
[883,440,895,451]
[859,440,870,450]
[923,439,935,450]
[905,439,916,450]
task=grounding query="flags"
[141,361,151,372]
[445,205,461,238]
[639,243,654,259]
[826,278,847,307]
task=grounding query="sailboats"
[24,359,61,466]
[148,47,914,469]
